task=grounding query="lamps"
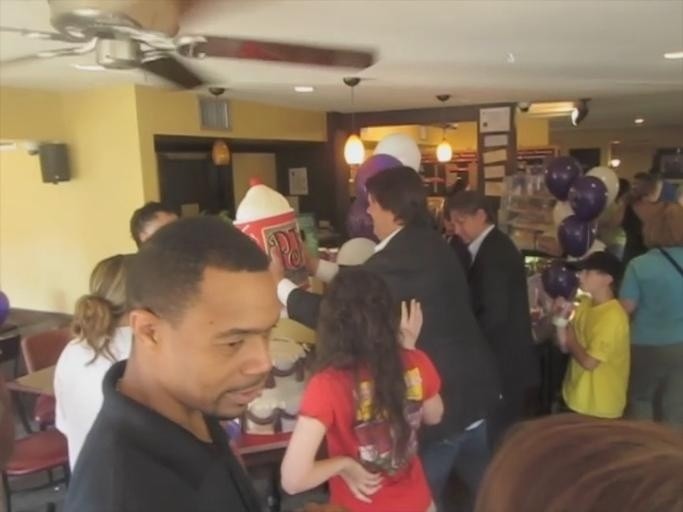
[436,94,452,162]
[344,77,364,165]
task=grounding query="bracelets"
[552,316,568,326]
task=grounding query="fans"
[0,0,377,90]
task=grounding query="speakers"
[39,142,72,184]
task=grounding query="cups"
[550,302,574,328]
[529,307,542,325]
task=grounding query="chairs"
[21,328,74,483]
[0,374,70,512]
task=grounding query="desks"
[232,432,294,512]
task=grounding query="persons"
[59,212,282,510]
[599,170,680,422]
[473,412,682,512]
[438,189,541,421]
[271,167,499,503]
[52,201,182,478]
[278,266,445,511]
[551,251,630,420]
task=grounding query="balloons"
[538,154,621,255]
[544,263,577,300]
[333,129,423,268]
[538,154,621,301]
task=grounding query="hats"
[565,251,621,271]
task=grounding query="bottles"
[235,176,308,291]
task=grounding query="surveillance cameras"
[517,100,532,113]
